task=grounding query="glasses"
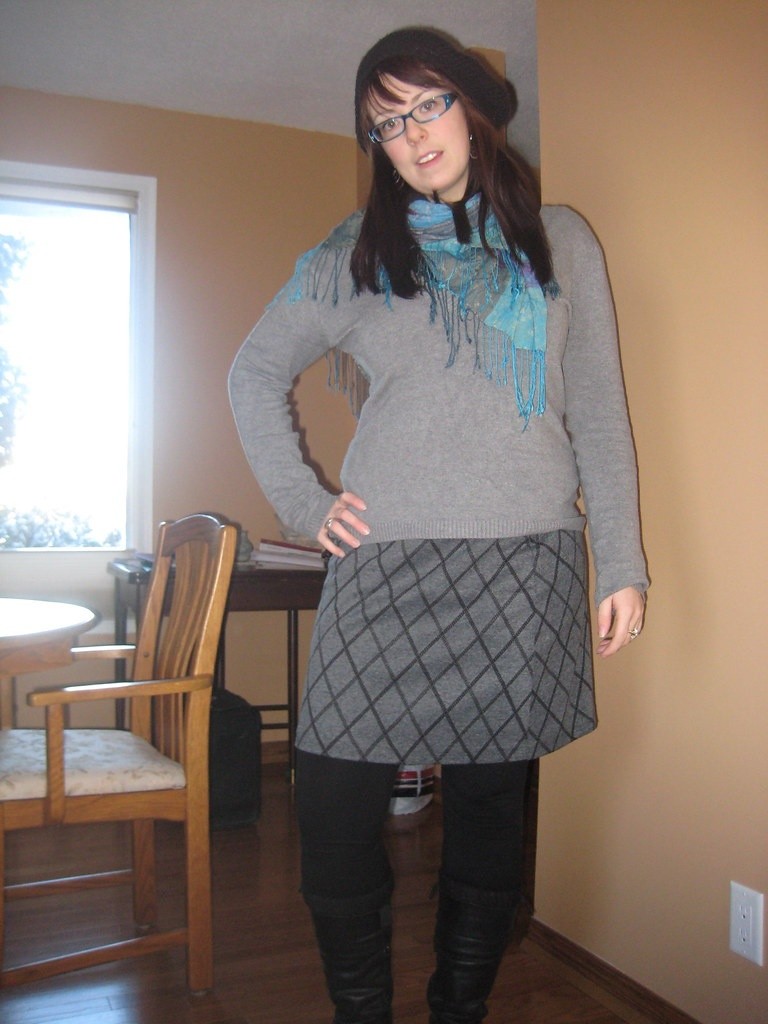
[367,93,458,144]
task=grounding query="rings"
[324,517,334,529]
[628,628,638,639]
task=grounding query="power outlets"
[728,881,765,966]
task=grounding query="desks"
[112,549,327,777]
[0,598,97,729]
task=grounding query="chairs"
[0,509,240,996]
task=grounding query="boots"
[301,878,394,1024]
[427,872,526,1024]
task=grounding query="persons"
[227,24,651,1024]
